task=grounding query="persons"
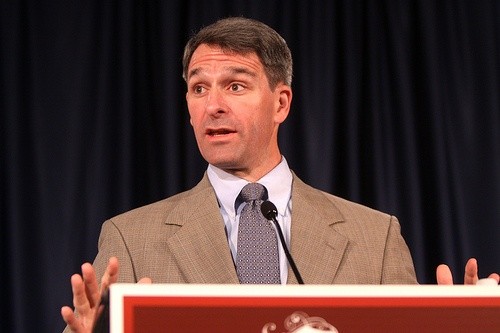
[60,19,499,333]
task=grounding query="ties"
[236,183,281,284]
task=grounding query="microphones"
[261,201,305,285]
